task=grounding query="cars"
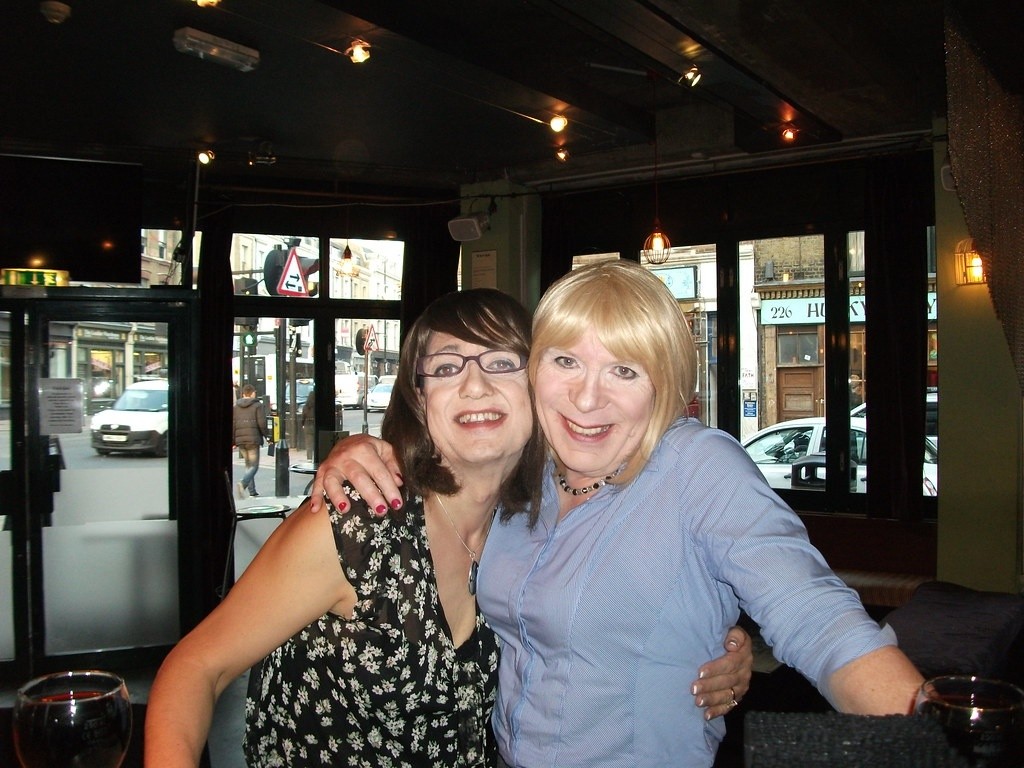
[743,414,939,497]
[358,374,379,410]
[848,386,939,436]
[367,375,397,412]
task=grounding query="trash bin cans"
[791,454,857,493]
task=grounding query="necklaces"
[559,461,627,496]
[432,489,499,596]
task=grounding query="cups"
[912,677,1024,768]
[17,670,132,768]
[267,444,274,456]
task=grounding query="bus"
[232,355,359,408]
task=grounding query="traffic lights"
[239,324,257,347]
[290,256,320,326]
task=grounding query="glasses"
[415,349,529,378]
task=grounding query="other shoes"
[249,491,261,497]
[236,481,246,498]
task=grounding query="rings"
[730,688,739,707]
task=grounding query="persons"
[310,259,938,768]
[233,385,269,497]
[145,288,755,766]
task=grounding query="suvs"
[90,379,169,457]
